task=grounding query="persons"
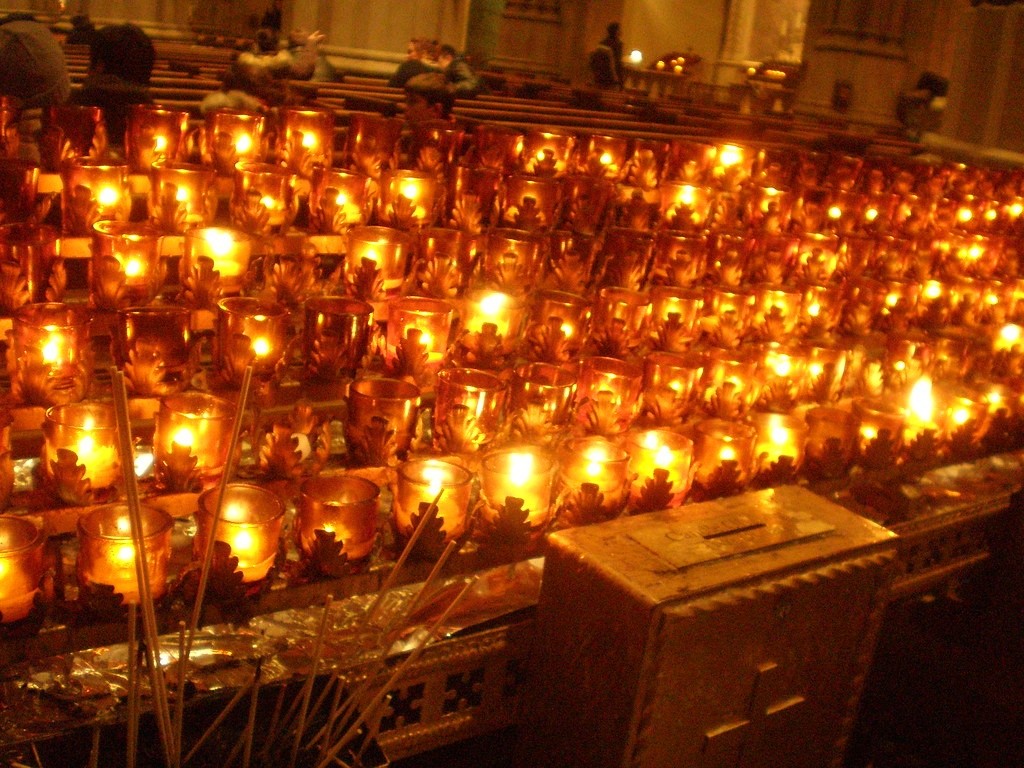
[589,22,624,91]
[277,27,344,83]
[72,25,155,145]
[0,20,71,111]
[386,36,457,94]
[436,43,489,96]
[220,28,294,110]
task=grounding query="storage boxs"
[514,485,903,768]
